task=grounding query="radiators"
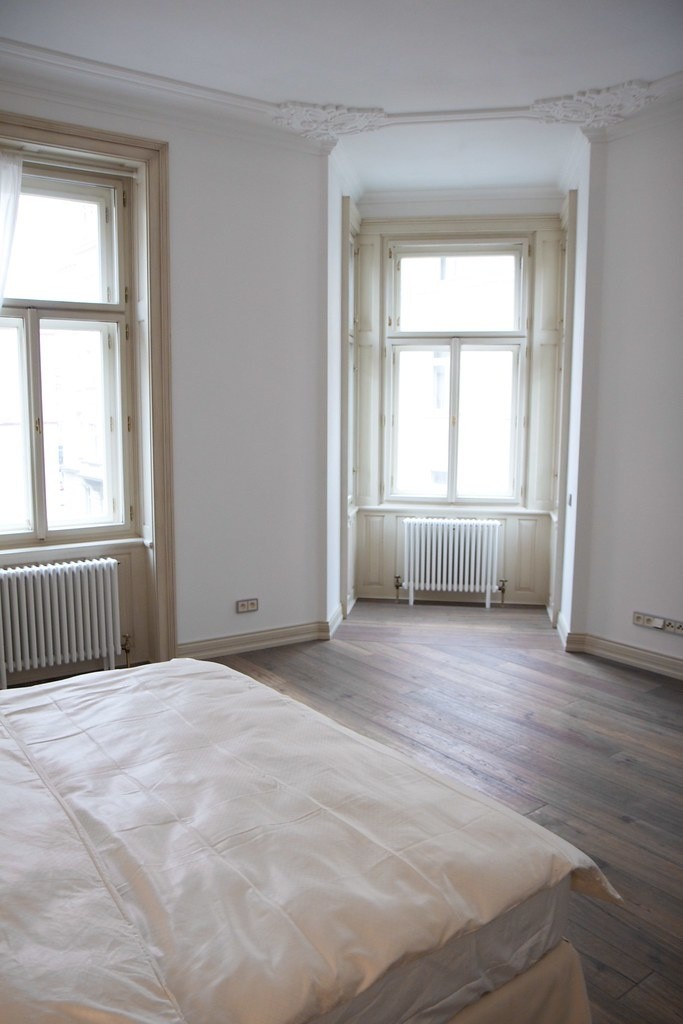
[402,516,502,609]
[1,557,122,690]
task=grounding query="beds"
[1,658,625,1024]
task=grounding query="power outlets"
[664,619,674,633]
[236,600,248,613]
[643,615,653,629]
[675,621,683,636]
[633,611,644,625]
[653,617,665,631]
[247,599,258,612]
